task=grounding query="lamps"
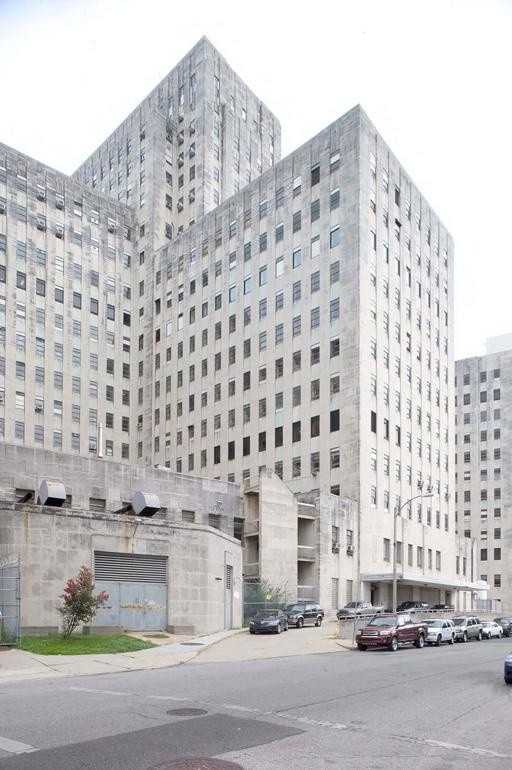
[129,516,142,536]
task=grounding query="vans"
[396,600,430,614]
[430,604,456,613]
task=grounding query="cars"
[355,614,512,651]
[504,652,512,685]
[247,607,288,636]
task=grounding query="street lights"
[392,492,433,612]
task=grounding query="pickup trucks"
[337,600,385,619]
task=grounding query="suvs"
[283,601,325,629]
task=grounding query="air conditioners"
[347,545,355,554]
[56,199,64,209]
[333,543,340,549]
[0,203,4,212]
[36,220,46,229]
[56,227,63,238]
[36,191,45,201]
[108,224,116,232]
